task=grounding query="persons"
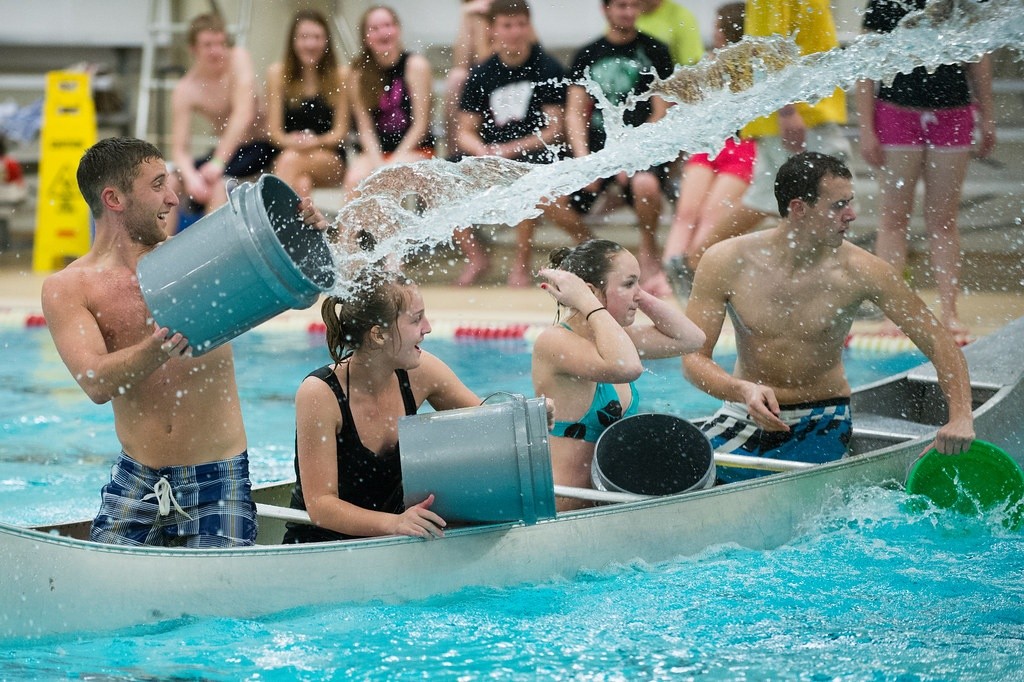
[532,239,705,512]
[41,137,328,547]
[681,152,975,484]
[854,0,997,342]
[168,0,845,286]
[280,275,555,546]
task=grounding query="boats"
[0,313,1024,646]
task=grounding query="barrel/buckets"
[903,440,1024,539]
[134,175,336,356]
[590,413,716,506]
[397,393,557,526]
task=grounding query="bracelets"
[587,307,607,319]
